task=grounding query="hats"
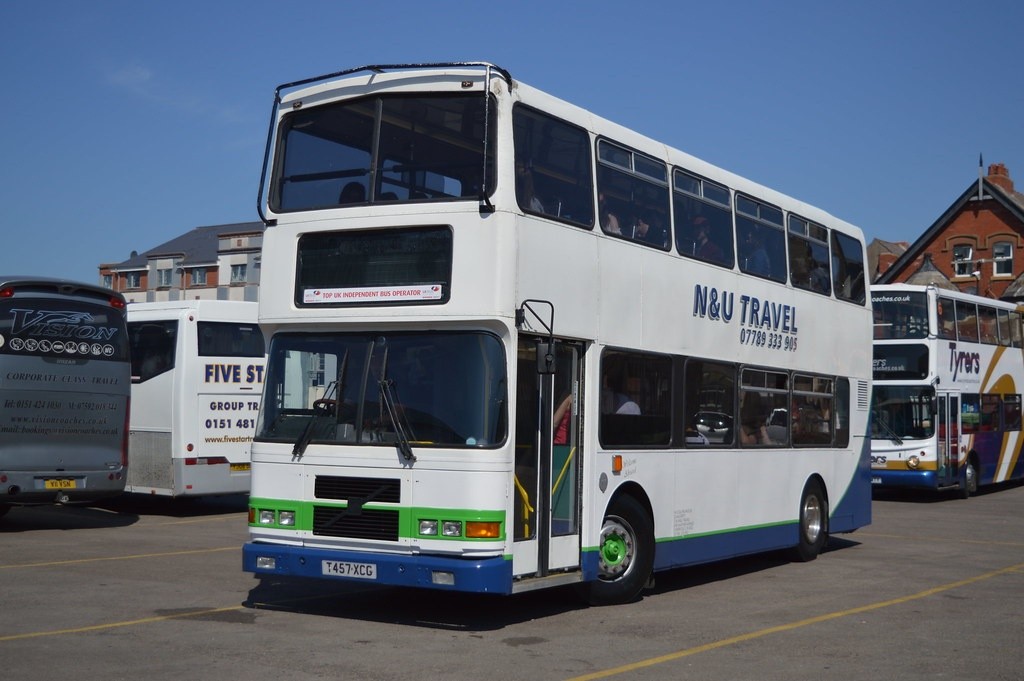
[691,217,710,225]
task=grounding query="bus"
[871,283,1024,499]
[241,61,874,603]
[124,300,309,500]
[0,275,132,517]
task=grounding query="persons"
[634,215,665,245]
[598,192,622,235]
[554,393,571,445]
[516,166,545,215]
[738,422,758,444]
[691,216,725,262]
